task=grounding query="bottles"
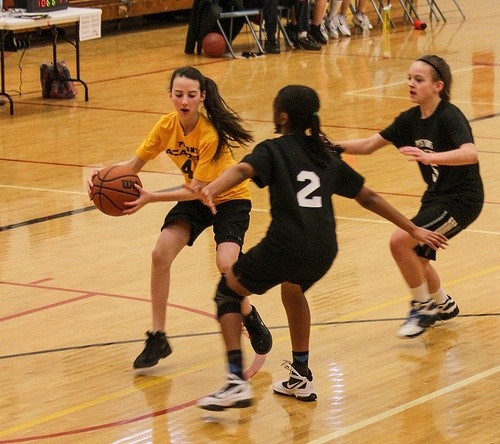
[382,5,390,33]
[414,21,427,31]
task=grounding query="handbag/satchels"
[39,60,78,99]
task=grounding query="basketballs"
[203,33,226,57]
[90,165,141,216]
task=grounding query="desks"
[0,7,102,118]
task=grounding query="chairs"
[184,0,465,58]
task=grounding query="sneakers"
[243,304,273,355]
[195,372,254,412]
[396,296,441,336]
[437,295,460,320]
[132,328,172,368]
[272,360,318,401]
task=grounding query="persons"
[246,0,376,56]
[80,66,275,371]
[331,52,485,339]
[197,83,448,412]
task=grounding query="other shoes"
[309,23,327,45]
[320,25,329,41]
[336,13,351,37]
[293,30,322,50]
[323,16,339,38]
[351,13,373,30]
[265,37,280,54]
